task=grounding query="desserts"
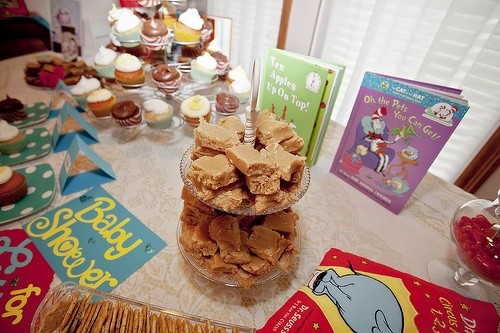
[0,94,26,122]
[0,119,28,155]
[0,166,27,205]
[70,0,239,130]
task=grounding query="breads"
[179,184,297,283]
[24,56,94,86]
[186,109,308,213]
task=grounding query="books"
[257,46,346,167]
[328,70,470,216]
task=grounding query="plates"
[25,81,76,90]
[0,125,52,167]
[0,162,57,226]
[10,101,50,128]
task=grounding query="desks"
[0,44,500,333]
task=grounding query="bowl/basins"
[32,281,256,333]
